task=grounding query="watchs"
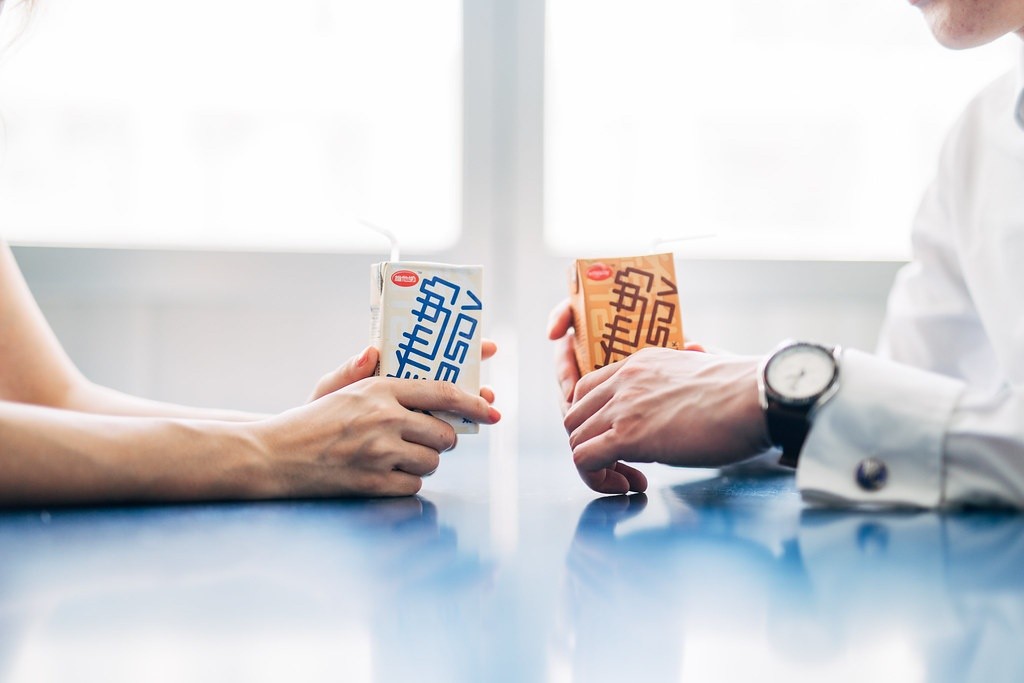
[758,339,838,471]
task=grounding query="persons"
[549,2,1023,512]
[0,238,500,512]
